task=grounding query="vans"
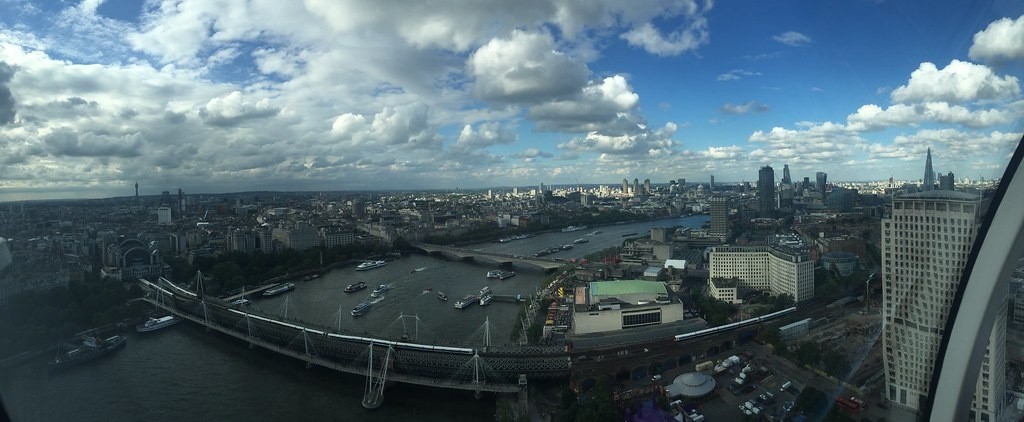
[780,381,792,391]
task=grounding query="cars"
[714,355,752,384]
[760,394,767,400]
[738,399,763,416]
[783,401,794,411]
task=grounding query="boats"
[136,315,185,332]
[475,287,489,303]
[370,285,389,297]
[573,238,588,244]
[562,225,588,232]
[356,260,388,271]
[533,244,573,257]
[480,295,492,305]
[48,334,127,368]
[263,282,295,296]
[427,287,432,291]
[454,295,474,309]
[487,269,517,281]
[409,269,415,274]
[350,302,372,316]
[436,291,448,301]
[344,281,367,292]
[592,230,602,235]
[622,232,638,237]
[499,232,540,242]
